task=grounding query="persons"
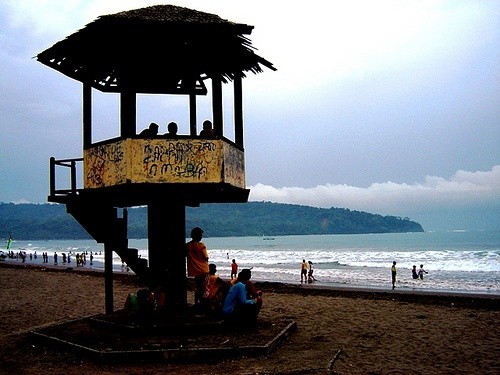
[230,269,263,303]
[301,259,307,281]
[418,264,428,280]
[231,259,238,280]
[185,227,210,302]
[392,261,396,290]
[202,263,231,300]
[225,269,261,324]
[164,122,179,136]
[140,123,160,136]
[226,253,229,259]
[0,249,141,271]
[308,261,317,282]
[412,265,418,279]
[199,120,214,137]
